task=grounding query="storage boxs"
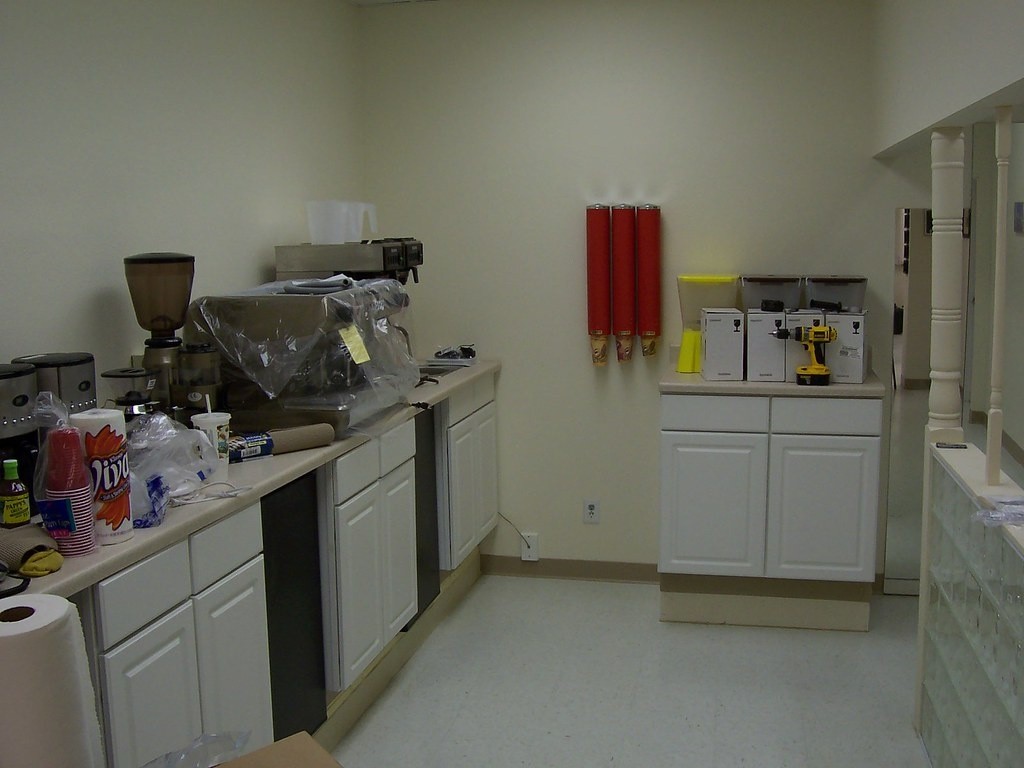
[700,305,868,386]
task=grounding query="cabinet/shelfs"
[330,417,416,506]
[334,457,419,691]
[92,498,265,652]
[440,370,495,428]
[661,392,883,438]
[97,554,274,768]
[446,399,500,572]
[657,430,881,584]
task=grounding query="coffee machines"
[275,238,423,286]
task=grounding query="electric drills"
[768,325,838,386]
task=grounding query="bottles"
[0,459,31,528]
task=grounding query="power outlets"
[522,532,539,561]
[583,497,600,524]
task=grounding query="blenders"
[806,275,869,315]
[674,276,739,372]
[125,252,194,384]
[101,367,161,423]
[741,275,803,312]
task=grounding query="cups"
[46,428,94,557]
[191,412,231,483]
